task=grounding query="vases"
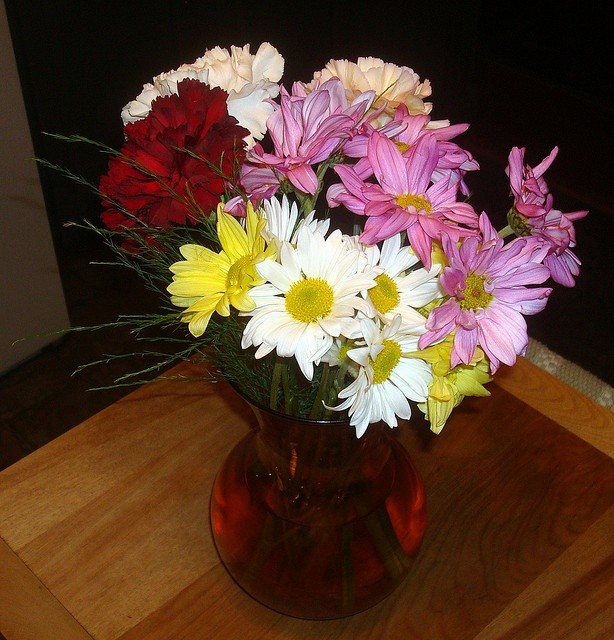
[208,375,429,621]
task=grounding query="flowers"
[8,41,588,440]
